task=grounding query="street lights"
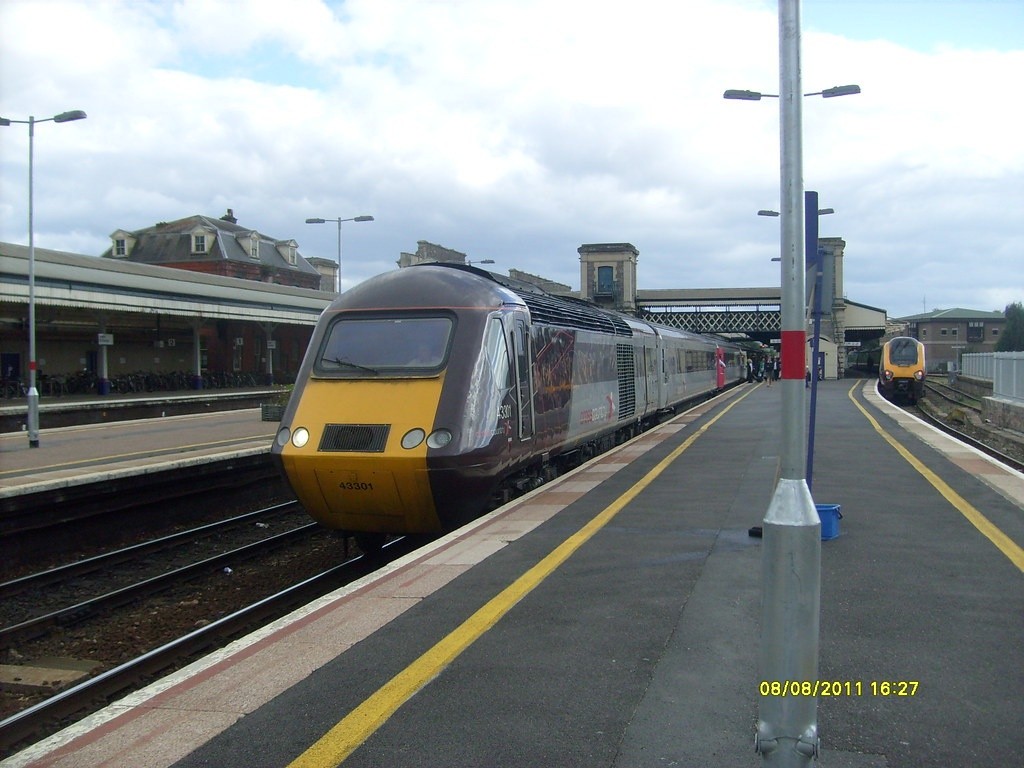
[0,109,88,448]
[305,214,375,295]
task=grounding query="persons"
[410,343,439,365]
[805,366,809,388]
[747,356,781,387]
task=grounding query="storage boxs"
[815,503,843,542]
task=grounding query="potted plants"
[260,384,295,422]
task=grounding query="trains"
[270,261,774,539]
[878,336,928,406]
